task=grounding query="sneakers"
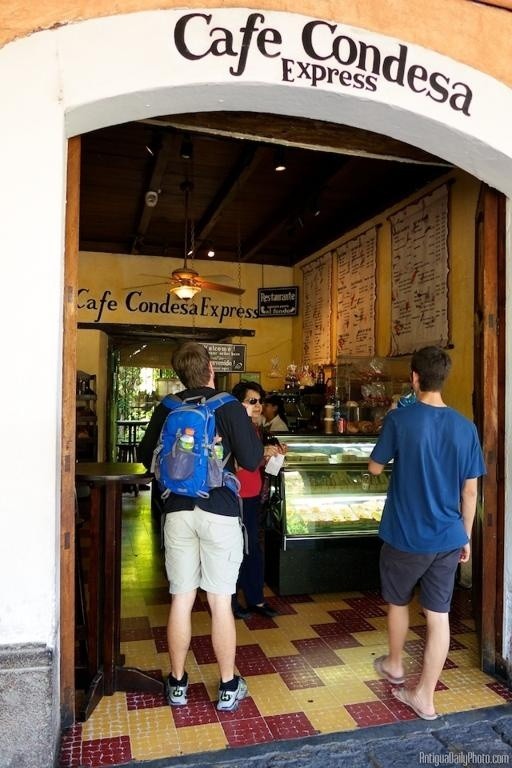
[166,670,189,706]
[217,676,247,711]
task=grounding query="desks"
[115,419,150,463]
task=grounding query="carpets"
[59,585,511,768]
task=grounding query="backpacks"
[153,391,239,499]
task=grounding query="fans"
[120,181,244,296]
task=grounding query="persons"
[259,396,289,434]
[368,346,488,720]
[228,382,288,619]
[136,342,264,712]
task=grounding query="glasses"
[245,397,263,405]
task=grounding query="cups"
[323,418,335,434]
[323,404,334,418]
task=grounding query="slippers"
[375,654,406,684]
[392,687,437,720]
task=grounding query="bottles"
[177,428,195,452]
[317,368,324,384]
[210,437,224,463]
[398,393,417,408]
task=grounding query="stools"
[115,444,137,463]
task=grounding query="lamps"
[169,285,201,300]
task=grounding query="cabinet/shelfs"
[77,369,97,463]
[263,431,420,596]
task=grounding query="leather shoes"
[233,606,249,619]
[249,603,277,617]
